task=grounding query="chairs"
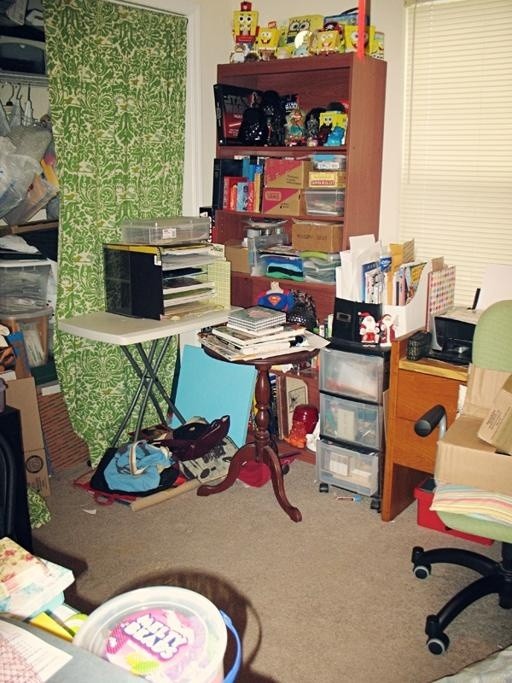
[407,301,512,655]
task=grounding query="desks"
[196,326,318,521]
[58,307,241,498]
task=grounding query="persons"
[358,312,400,348]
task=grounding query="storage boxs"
[123,215,210,245]
[1,260,52,372]
[255,149,347,284]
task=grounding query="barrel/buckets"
[75,587,243,683]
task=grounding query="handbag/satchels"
[74,417,230,506]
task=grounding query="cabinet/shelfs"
[316,337,383,499]
[205,52,389,463]
[382,325,473,525]
[103,240,226,319]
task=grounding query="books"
[212,153,269,212]
[195,303,308,365]
[163,277,217,308]
[0,533,78,625]
